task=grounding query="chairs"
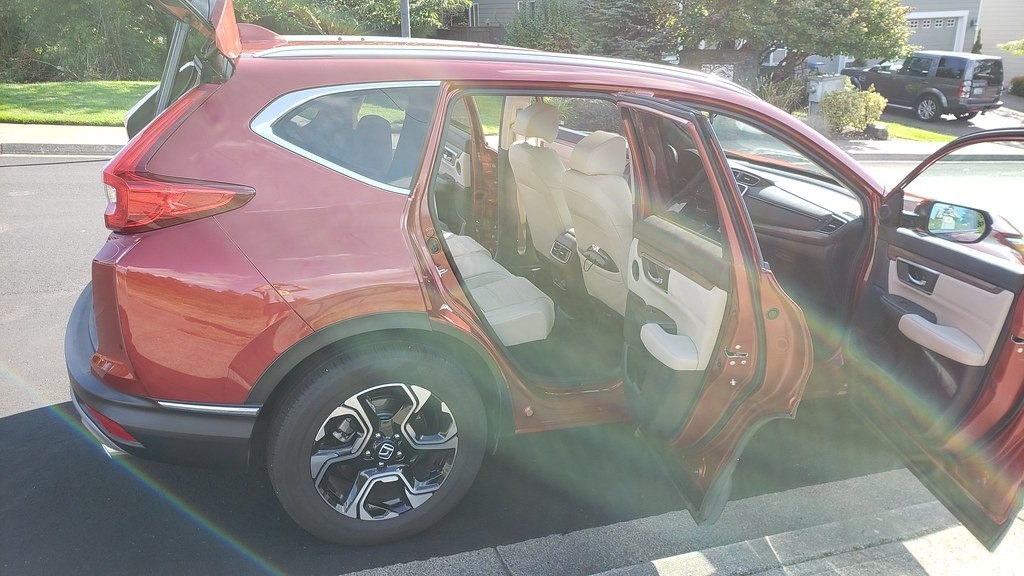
[445,235,493,258]
[470,276,555,347]
[454,252,512,289]
[350,115,392,173]
[560,130,633,316]
[508,102,576,269]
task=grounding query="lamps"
[970,17,980,35]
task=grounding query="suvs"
[840,50,1004,122]
[61,0,1023,556]
